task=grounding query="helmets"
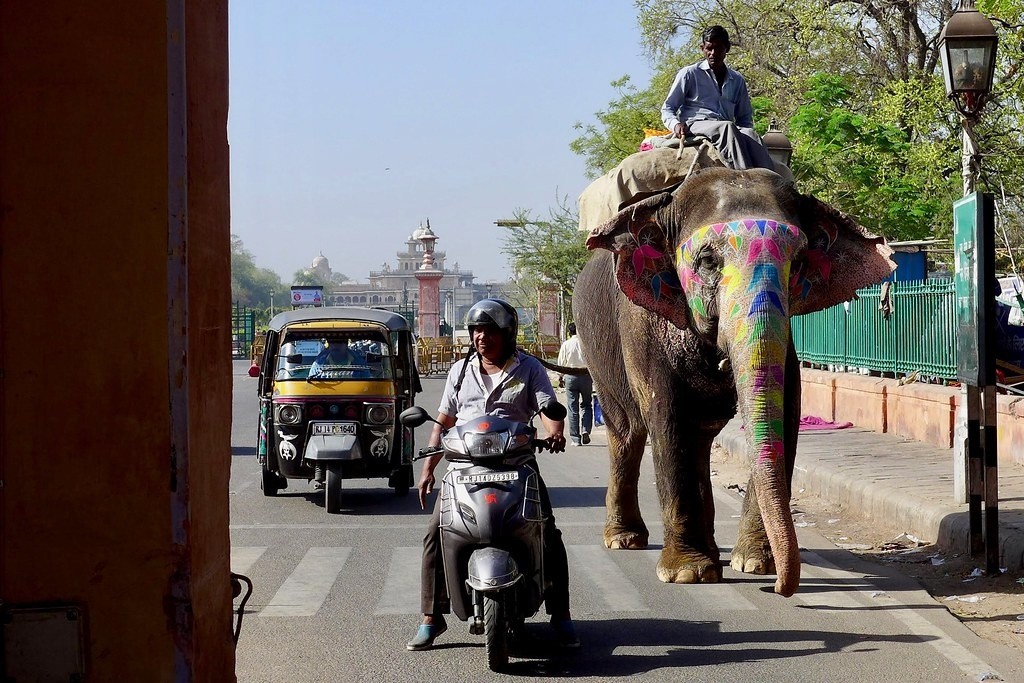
[467,298,519,349]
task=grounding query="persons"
[306,338,371,378]
[661,25,797,190]
[405,297,579,651]
[558,323,593,446]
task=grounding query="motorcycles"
[398,396,567,675]
[254,306,418,516]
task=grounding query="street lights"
[269,287,276,321]
[486,281,492,299]
[932,0,1003,577]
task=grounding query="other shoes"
[406,617,448,651]
[550,618,580,648]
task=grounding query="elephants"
[571,165,898,597]
